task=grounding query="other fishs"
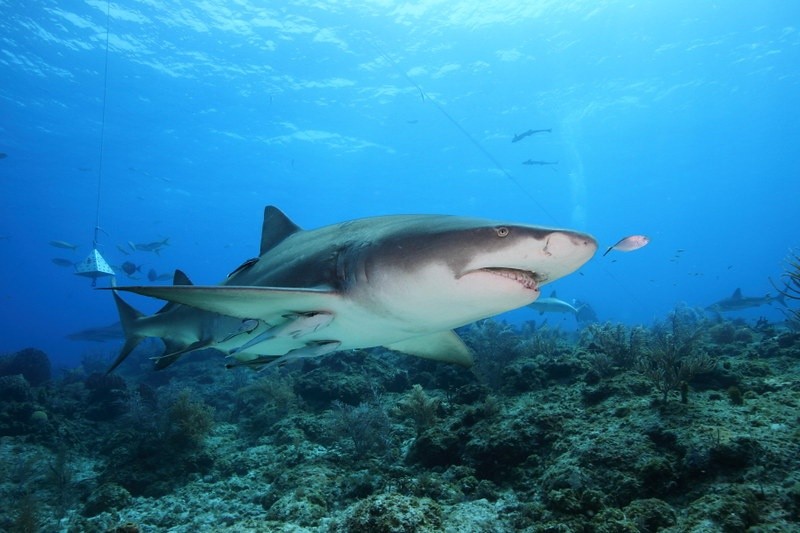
[49,127,653,322]
